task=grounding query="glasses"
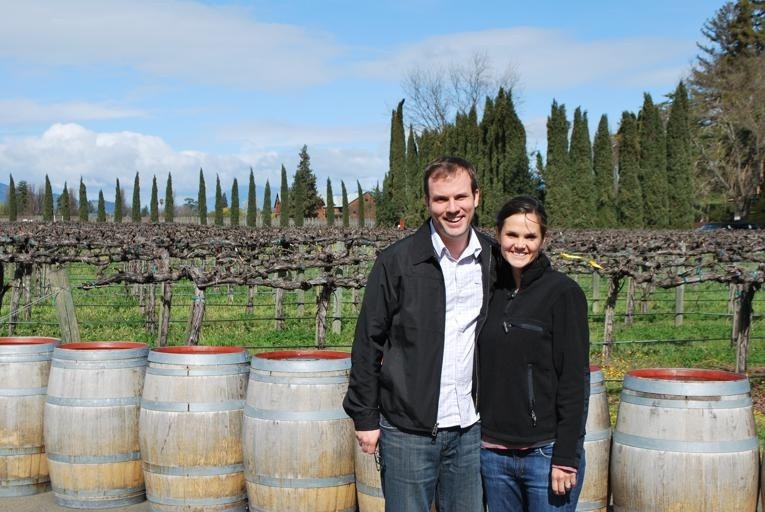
[375,450,385,471]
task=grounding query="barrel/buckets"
[40,341,149,509]
[352,438,440,512]
[576,365,612,512]
[137,346,250,512]
[610,368,759,512]
[0,336,62,498]
[242,350,363,512]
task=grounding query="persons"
[341,157,507,511]
[465,191,596,512]
